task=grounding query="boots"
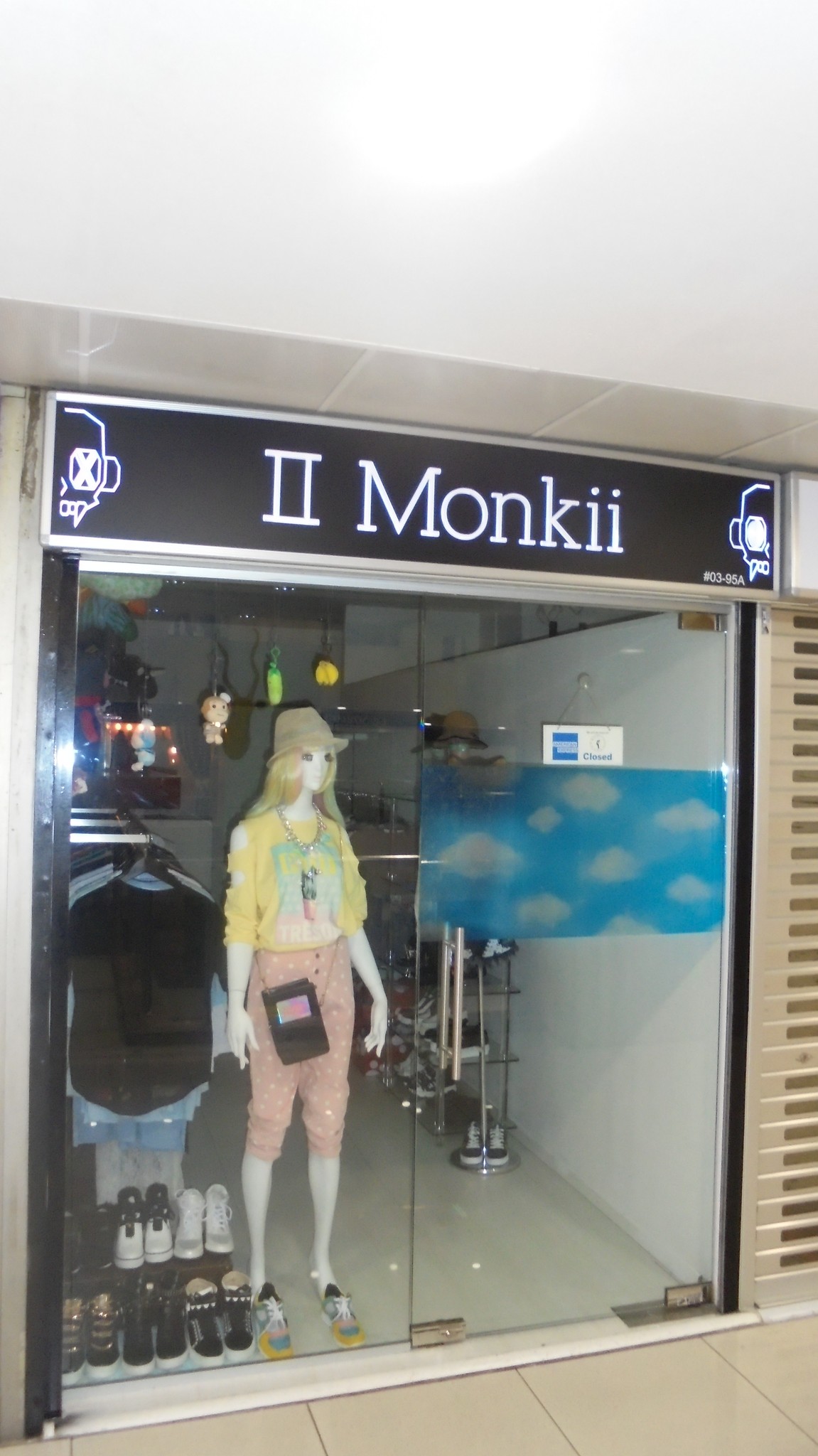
[124,1264,158,1367]
[155,1267,188,1359]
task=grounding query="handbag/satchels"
[263,976,331,1065]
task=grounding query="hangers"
[67,808,223,919]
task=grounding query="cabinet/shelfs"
[378,791,521,1146]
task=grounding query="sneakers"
[383,936,515,1098]
[173,1187,205,1259]
[251,1280,294,1360]
[144,1182,174,1264]
[321,1285,367,1349]
[114,1186,145,1270]
[201,1183,235,1255]
[458,1120,485,1165]
[87,1282,121,1367]
[59,1286,87,1373]
[486,1121,510,1166]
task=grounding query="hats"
[265,706,347,769]
[432,711,488,751]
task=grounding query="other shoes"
[62,1209,85,1275]
[184,1278,223,1358]
[85,1203,119,1269]
[217,1271,254,1350]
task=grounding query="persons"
[221,708,388,1360]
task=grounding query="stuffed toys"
[131,718,155,771]
[200,693,231,744]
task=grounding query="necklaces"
[274,801,326,852]
